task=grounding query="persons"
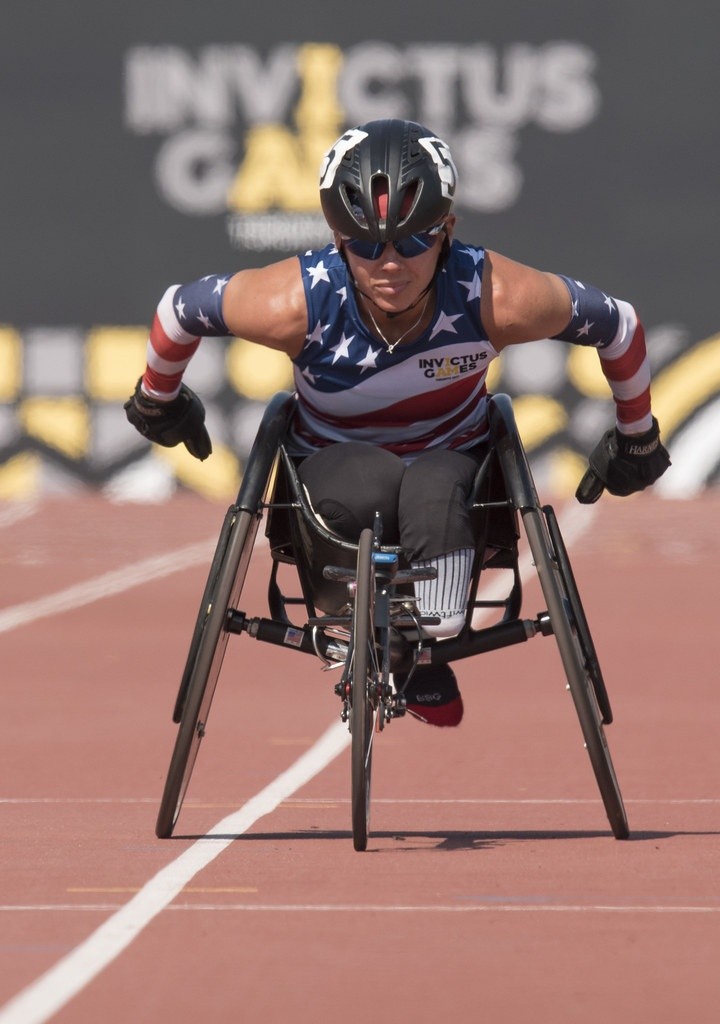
[125,118,672,726]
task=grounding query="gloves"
[124,375,212,461]
[575,415,670,503]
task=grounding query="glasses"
[337,221,447,261]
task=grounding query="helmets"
[319,118,458,242]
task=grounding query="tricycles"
[153,393,635,852]
